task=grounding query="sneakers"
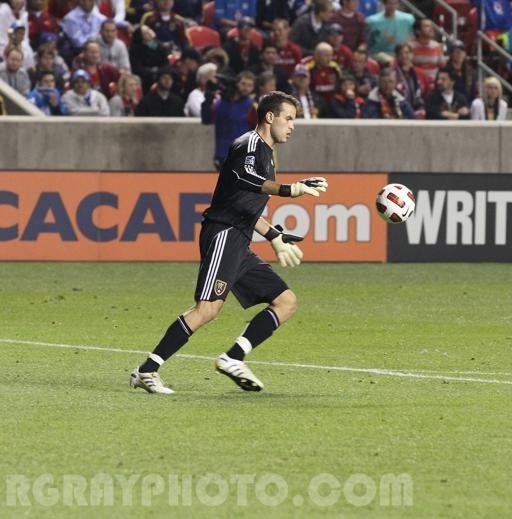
[128,365,176,396]
[213,351,266,392]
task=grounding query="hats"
[174,48,202,63]
[291,62,312,79]
[10,19,27,32]
[449,39,467,53]
[71,67,92,83]
[38,31,59,45]
[327,23,346,36]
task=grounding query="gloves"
[262,223,307,269]
[278,173,330,200]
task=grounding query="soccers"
[376,183,416,223]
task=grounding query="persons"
[201,76,258,173]
[130,90,329,393]
[0,0,512,120]
